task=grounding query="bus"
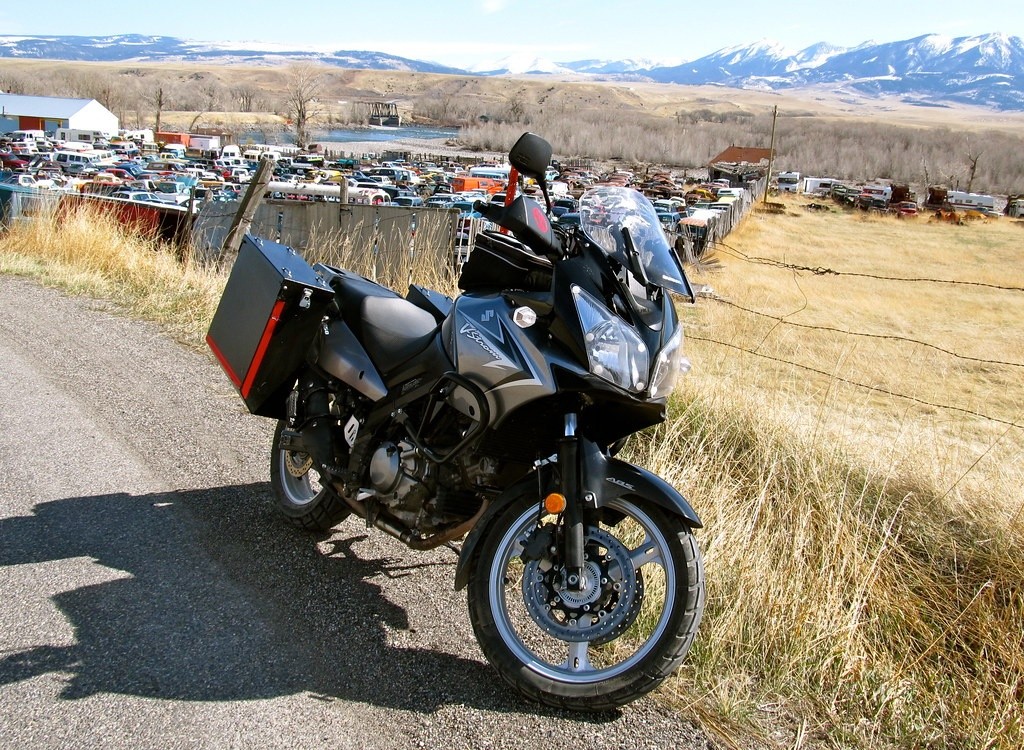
[777,171,800,193]
[944,190,995,214]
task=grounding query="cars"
[828,169,919,218]
[0,133,733,233]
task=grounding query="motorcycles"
[199,129,709,714]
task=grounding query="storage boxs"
[205,233,335,414]
